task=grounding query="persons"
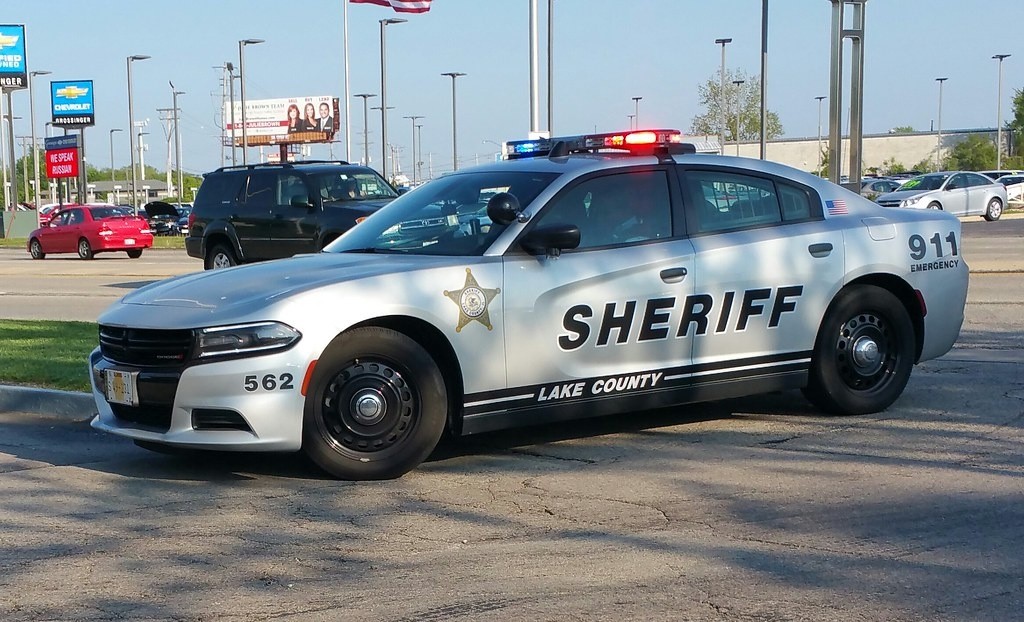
[592,180,655,243]
[301,104,318,132]
[287,104,303,134]
[317,102,333,132]
[343,178,367,198]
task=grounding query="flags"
[348,0,432,11]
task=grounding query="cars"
[840,169,1024,222]
[87,125,972,480]
[119,200,193,237]
[26,205,154,260]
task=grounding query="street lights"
[443,72,467,173]
[45,122,55,145]
[732,80,744,157]
[380,18,409,181]
[238,36,265,166]
[815,96,828,180]
[402,115,428,186]
[29,70,53,209]
[934,77,949,174]
[991,54,1013,169]
[627,114,635,130]
[110,53,153,217]
[632,96,643,131]
[353,93,376,167]
[232,73,241,168]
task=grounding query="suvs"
[184,159,463,273]
